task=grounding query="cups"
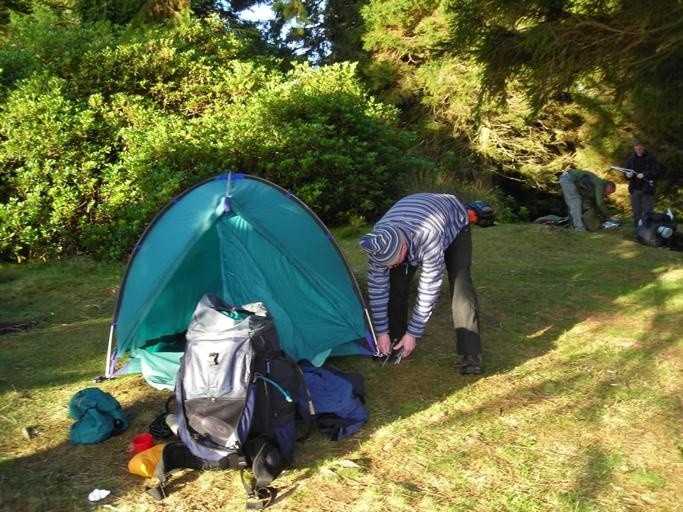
[656,225,673,239]
[131,433,153,451]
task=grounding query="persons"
[558,168,616,233]
[358,193,485,374]
[623,140,660,234]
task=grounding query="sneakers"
[372,341,403,360]
[461,355,483,374]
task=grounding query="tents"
[103,170,378,381]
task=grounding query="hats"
[360,222,402,264]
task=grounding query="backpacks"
[464,200,495,227]
[637,211,674,246]
[146,292,314,510]
